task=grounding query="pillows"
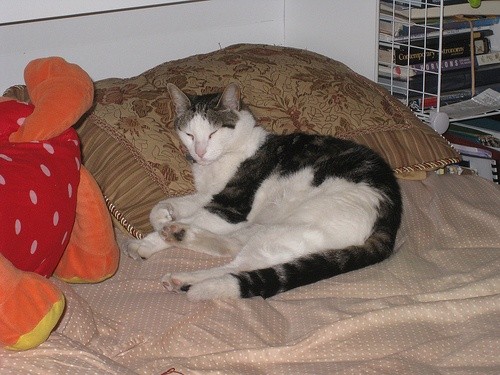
[4,44,462,238]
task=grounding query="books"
[379,0,498,107]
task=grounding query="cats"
[127,82,403,300]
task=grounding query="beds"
[0,44,500,374]
